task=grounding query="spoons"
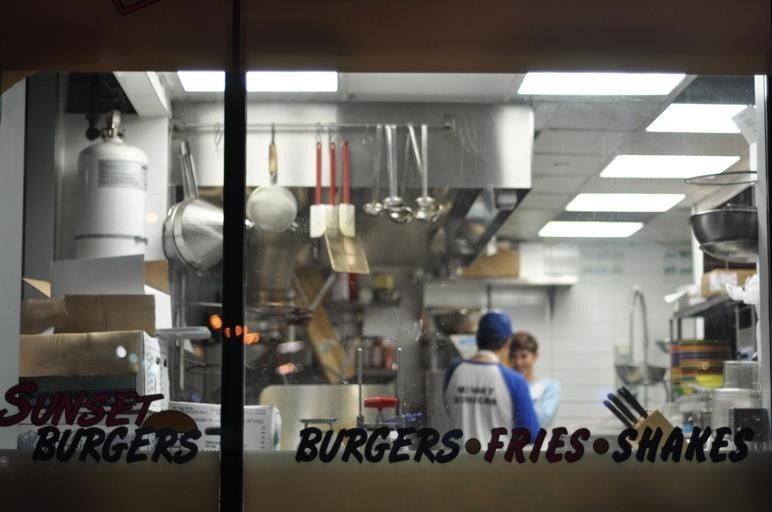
[412,122,440,226]
[406,121,447,225]
[385,129,416,224]
[362,124,387,218]
[381,124,407,212]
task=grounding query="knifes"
[617,388,647,418]
[603,399,632,430]
[606,393,638,424]
[621,385,649,419]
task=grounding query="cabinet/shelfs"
[671,289,761,362]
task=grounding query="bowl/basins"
[689,206,757,251]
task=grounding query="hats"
[476,311,512,346]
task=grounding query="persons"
[503,331,558,441]
[443,312,539,445]
